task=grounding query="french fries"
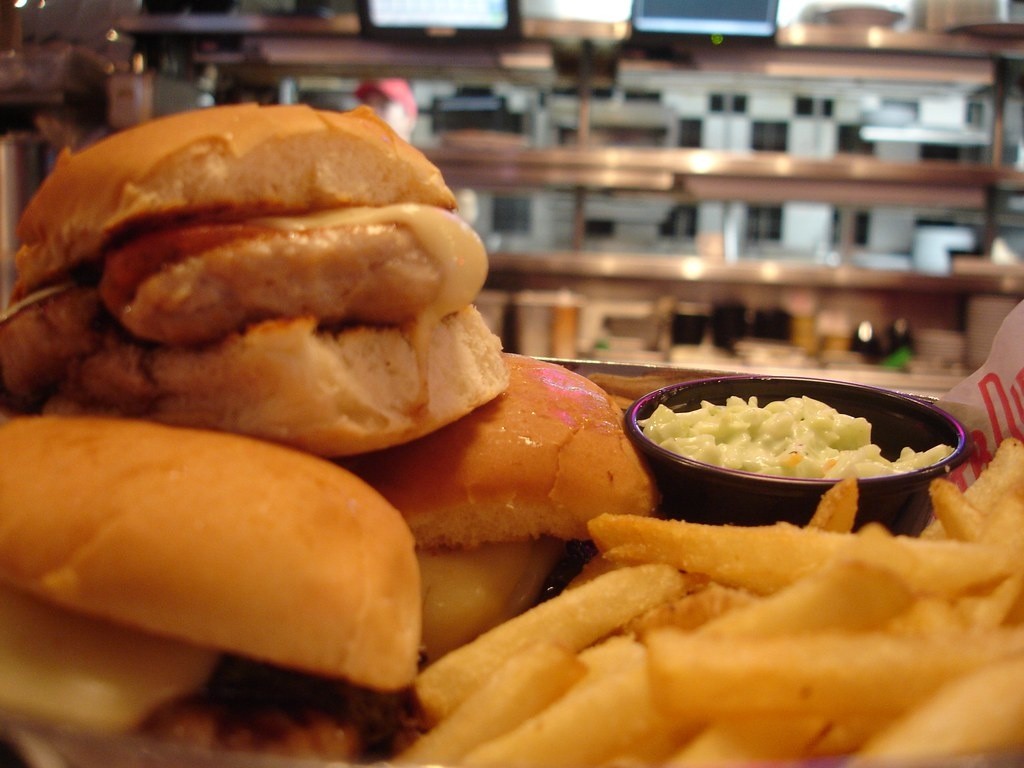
[381,434,1024,768]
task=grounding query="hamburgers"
[0,103,656,768]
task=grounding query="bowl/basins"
[624,375,965,537]
